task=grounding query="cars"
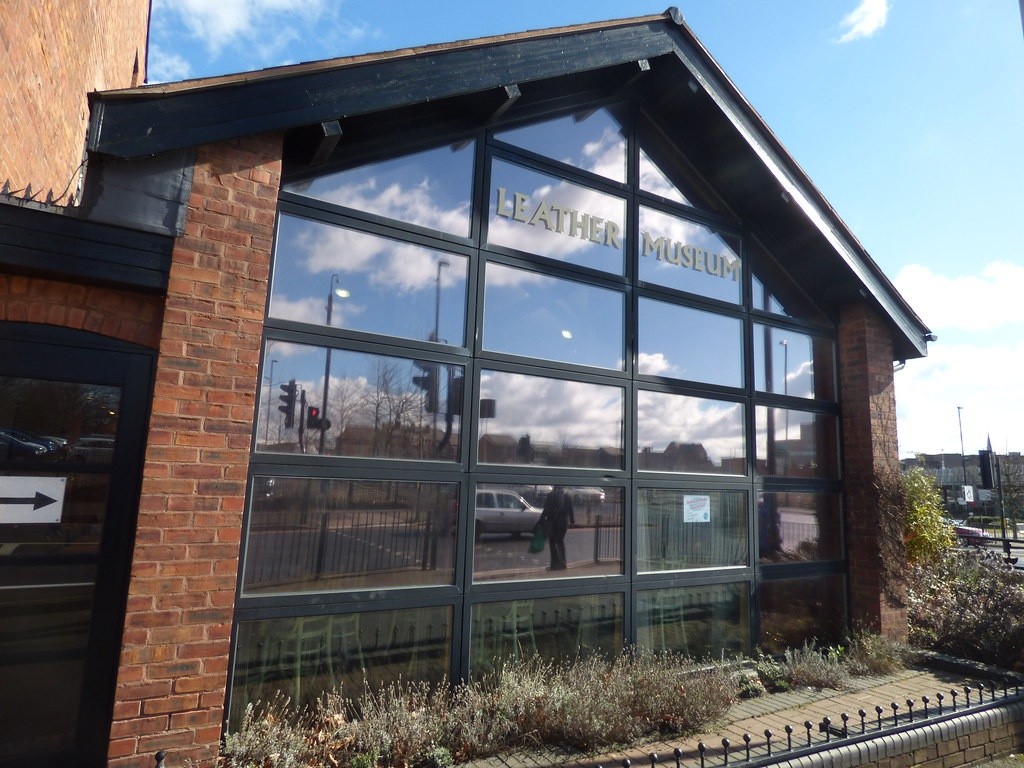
[448,489,545,538]
[565,484,605,504]
[520,483,553,498]
[941,518,989,547]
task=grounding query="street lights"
[265,359,278,445]
[432,259,450,454]
[780,340,789,508]
[957,406,967,486]
[318,273,350,455]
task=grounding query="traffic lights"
[308,406,319,427]
[278,379,295,429]
[412,330,438,412]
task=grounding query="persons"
[536,486,574,570]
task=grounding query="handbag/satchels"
[527,525,545,554]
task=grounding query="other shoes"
[546,566,566,571]
